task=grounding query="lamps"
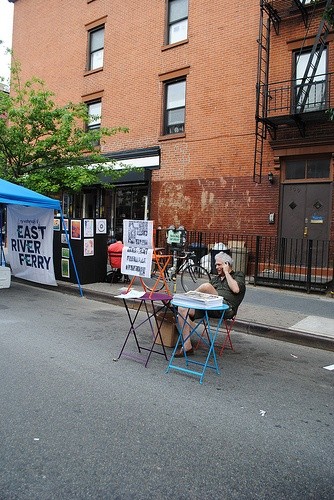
[268,172,274,183]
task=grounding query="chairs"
[195,309,238,356]
[125,229,174,299]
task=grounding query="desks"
[165,300,229,385]
[113,292,175,368]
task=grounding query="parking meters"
[167,225,188,294]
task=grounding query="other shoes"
[162,300,177,312]
[172,348,193,358]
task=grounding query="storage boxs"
[154,312,195,347]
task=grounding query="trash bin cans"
[211,248,230,274]
[231,248,247,275]
[248,262,257,274]
[190,245,208,261]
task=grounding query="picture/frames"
[84,219,94,237]
[61,233,69,244]
[62,219,69,231]
[71,219,81,240]
[96,219,107,234]
[62,248,70,259]
[61,259,70,278]
[53,219,61,231]
[83,238,94,256]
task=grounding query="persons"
[107,233,130,282]
[172,252,246,358]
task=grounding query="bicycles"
[140,247,212,293]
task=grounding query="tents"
[0,179,84,296]
